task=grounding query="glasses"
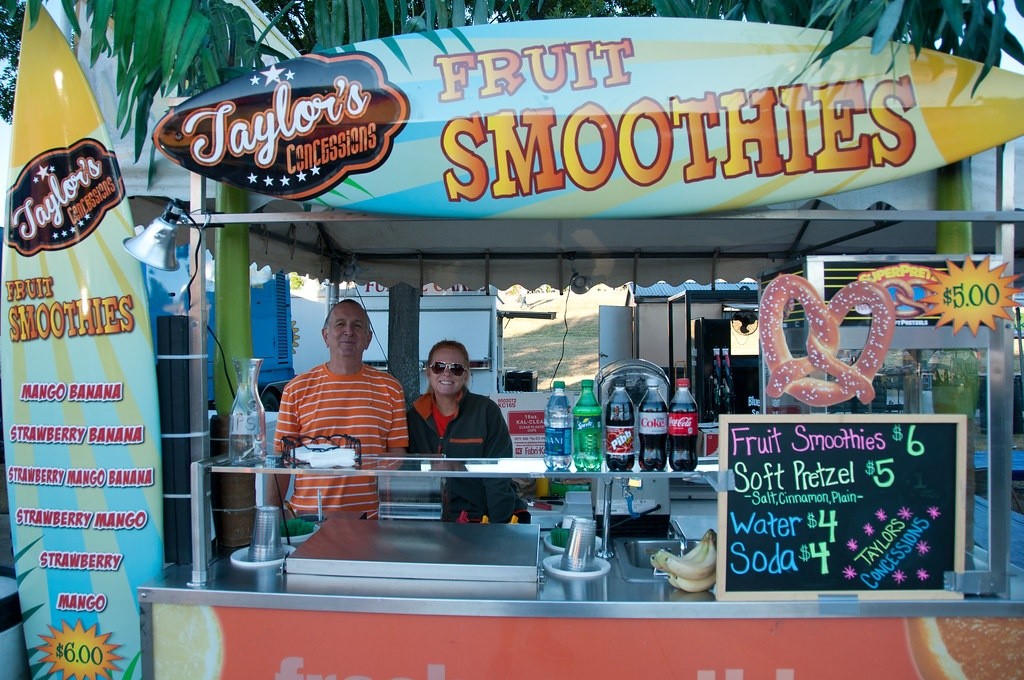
[429,361,467,377]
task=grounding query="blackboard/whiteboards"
[716,414,970,602]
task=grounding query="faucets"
[667,518,688,557]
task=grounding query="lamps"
[122,197,184,272]
[571,269,592,296]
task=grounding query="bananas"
[650,529,717,593]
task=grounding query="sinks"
[612,537,702,583]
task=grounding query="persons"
[391,340,528,524]
[265,299,408,521]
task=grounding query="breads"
[760,275,896,407]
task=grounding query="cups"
[247,506,284,563]
[560,516,597,572]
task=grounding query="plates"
[280,523,320,543]
[230,545,297,567]
[541,555,612,577]
[543,533,602,554]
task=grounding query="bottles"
[667,378,699,471]
[544,381,573,470]
[228,358,267,465]
[573,379,603,471]
[636,378,669,471]
[604,379,635,471]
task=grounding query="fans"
[732,311,758,336]
